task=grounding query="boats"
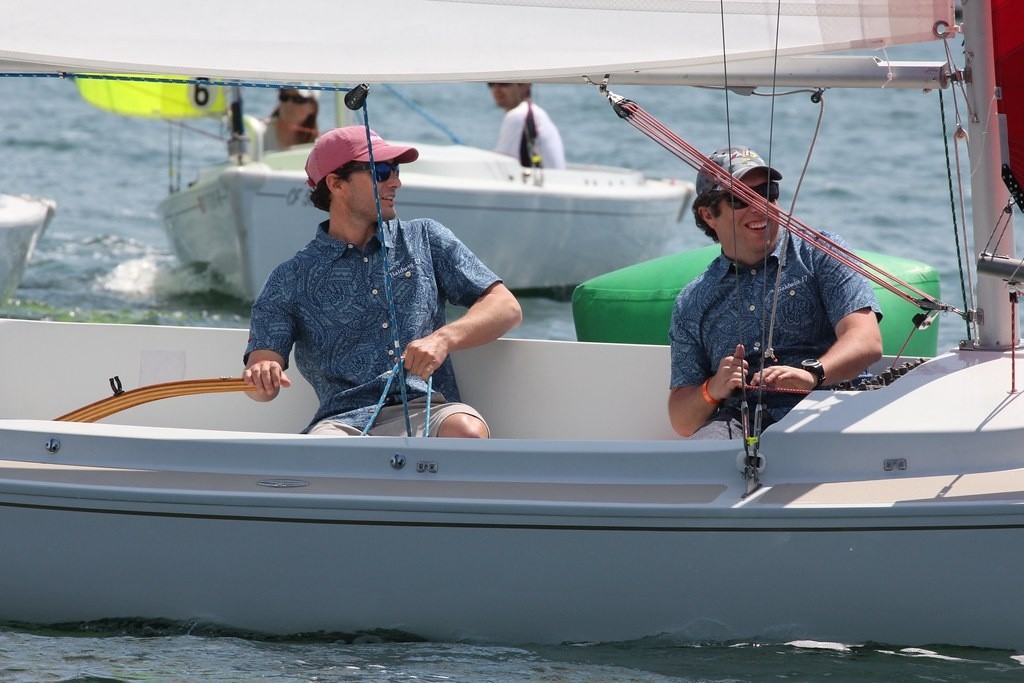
[0,188,54,282]
[153,137,699,306]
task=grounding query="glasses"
[279,95,306,104]
[337,159,399,182]
[709,182,779,209]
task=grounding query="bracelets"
[701,377,717,405]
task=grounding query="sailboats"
[0,4,1024,652]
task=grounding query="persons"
[240,124,523,438]
[668,146,884,438]
[263,84,320,152]
[489,83,564,169]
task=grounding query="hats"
[305,125,419,187]
[696,146,782,195]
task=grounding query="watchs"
[802,359,826,390]
